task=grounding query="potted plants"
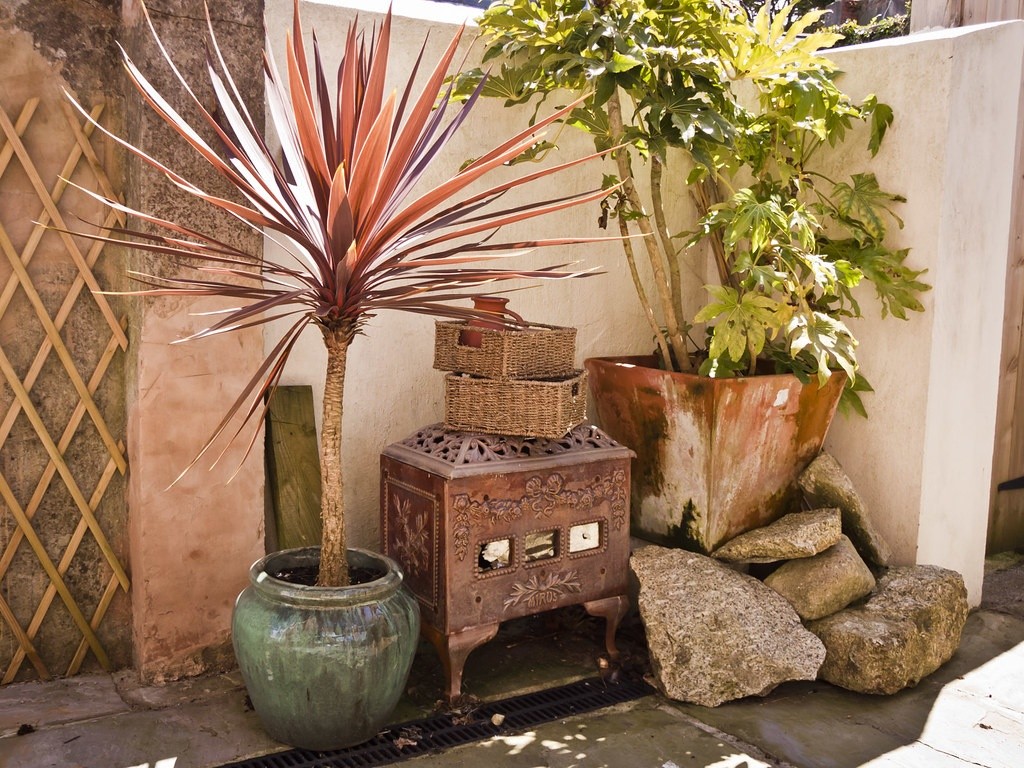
[432,1,932,552]
[32,0,654,752]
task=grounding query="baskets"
[443,368,590,440]
[432,319,579,381]
